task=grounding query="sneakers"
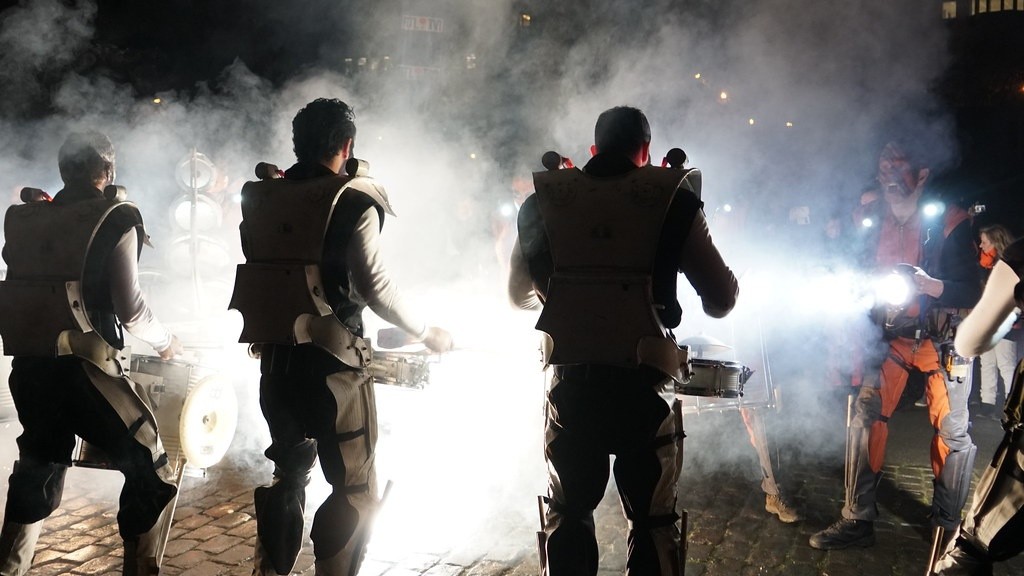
[932,526,956,546]
[766,491,807,523]
[809,517,876,549]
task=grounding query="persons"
[508,106,740,576]
[228,97,455,576]
[0,132,182,576]
[488,135,1024,576]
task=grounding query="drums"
[673,357,746,397]
[367,350,430,388]
[660,329,778,416]
[138,237,187,302]
[168,151,222,242]
[73,350,244,479]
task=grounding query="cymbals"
[179,378,239,469]
[677,334,733,354]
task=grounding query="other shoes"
[975,403,996,417]
[915,394,929,407]
[992,406,1004,421]
